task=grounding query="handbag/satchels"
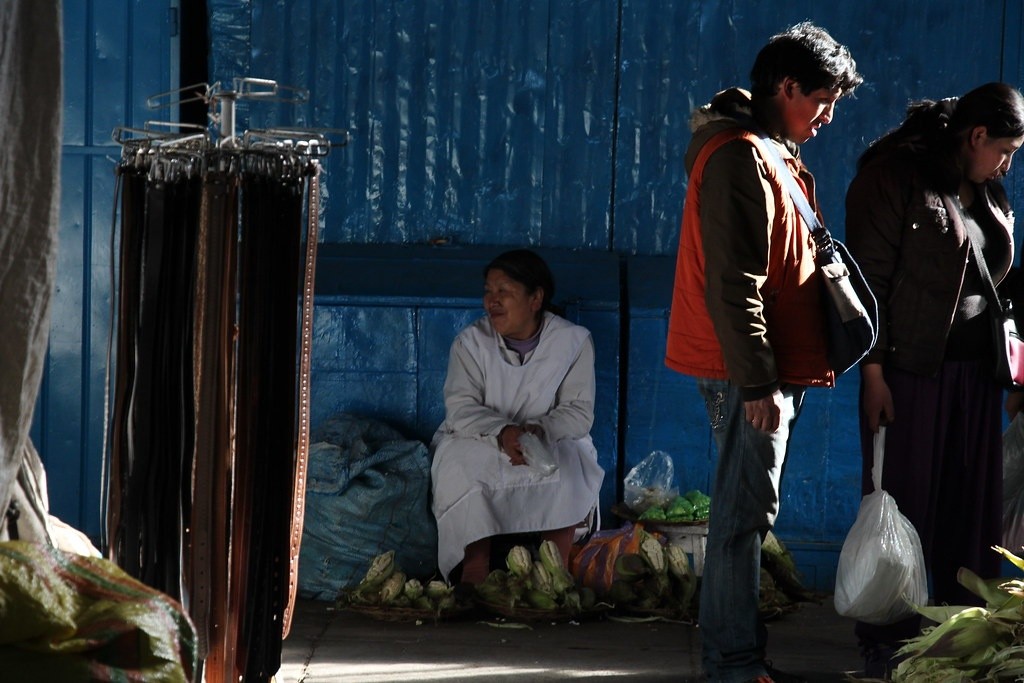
[816,241,879,377]
[994,301,1024,392]
[833,426,929,623]
[572,520,666,599]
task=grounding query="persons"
[841,82,1024,683]
[430,249,607,607]
[665,20,864,682]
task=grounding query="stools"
[651,518,710,576]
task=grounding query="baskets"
[481,595,560,621]
[349,598,435,623]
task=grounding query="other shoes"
[453,582,482,614]
